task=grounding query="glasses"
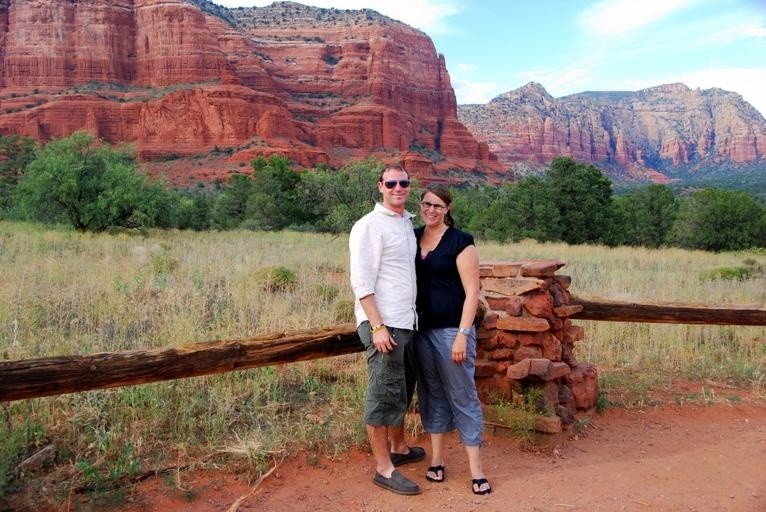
[382,179,410,190]
[418,199,447,211]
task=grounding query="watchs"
[457,326,471,335]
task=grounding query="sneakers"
[390,445,425,467]
[373,469,421,495]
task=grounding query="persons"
[347,166,429,494]
[413,185,493,494]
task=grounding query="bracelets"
[370,323,386,333]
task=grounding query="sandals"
[472,478,491,495]
[425,465,445,482]
